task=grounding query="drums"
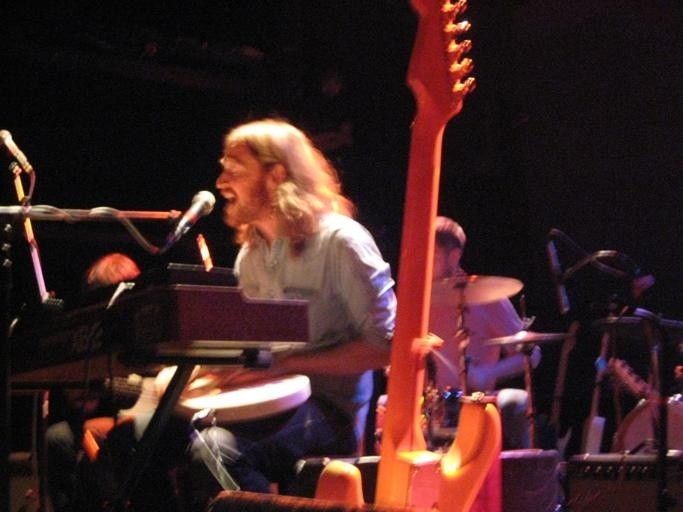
[374,386,463,457]
[613,393,683,452]
[554,450,682,512]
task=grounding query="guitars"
[316,0,504,512]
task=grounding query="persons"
[0,118,682,511]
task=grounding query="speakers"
[294,448,565,512]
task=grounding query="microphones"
[163,190,216,250]
[547,239,571,315]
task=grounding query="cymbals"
[430,275,525,305]
[482,330,574,345]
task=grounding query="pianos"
[0,263,310,396]
